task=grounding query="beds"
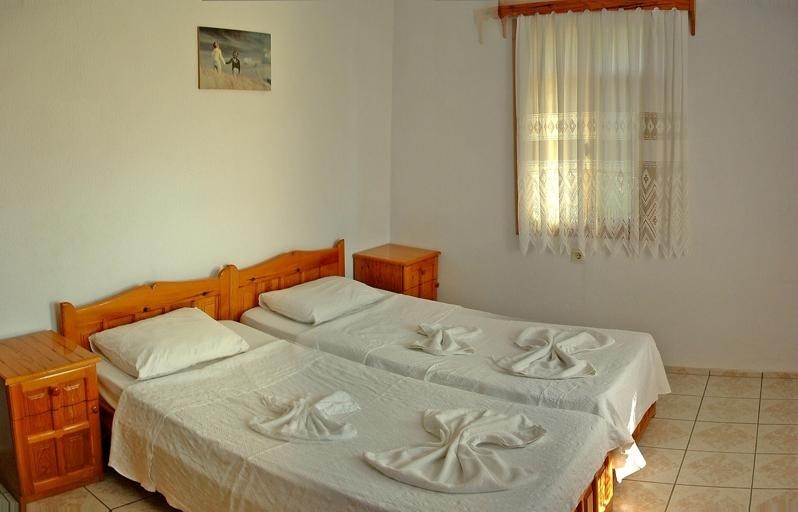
[227,240,671,485]
[58,263,638,512]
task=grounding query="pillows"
[257,272,386,325]
[90,301,250,381]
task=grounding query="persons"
[211,40,225,73]
[226,51,240,77]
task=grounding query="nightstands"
[2,331,104,501]
[351,242,442,301]
